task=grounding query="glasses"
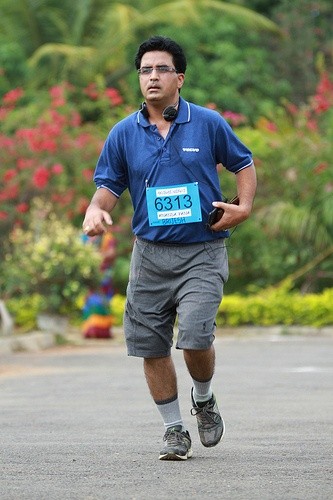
[137,66,177,73]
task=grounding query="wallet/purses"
[218,196,239,221]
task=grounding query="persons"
[82,36,257,459]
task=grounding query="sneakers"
[190,385,225,447]
[158,425,193,460]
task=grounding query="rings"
[85,226,89,230]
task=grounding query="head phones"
[138,99,181,121]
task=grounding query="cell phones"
[206,198,227,230]
